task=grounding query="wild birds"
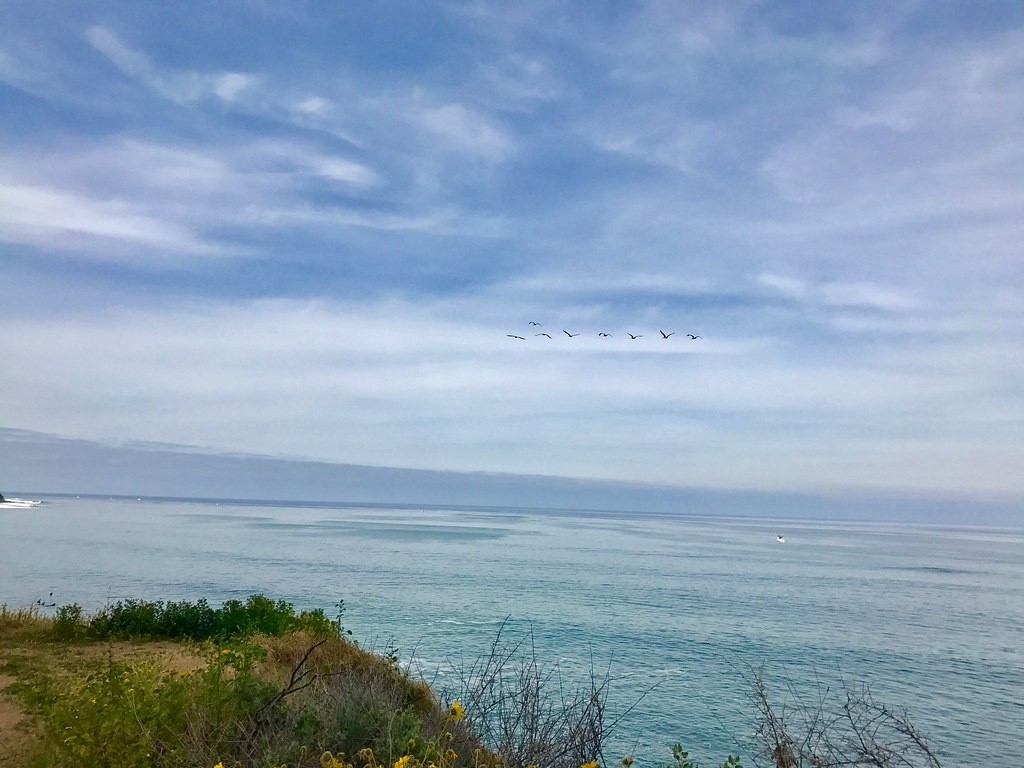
[506,320,701,344]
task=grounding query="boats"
[0,497,48,509]
[777,538,784,543]
